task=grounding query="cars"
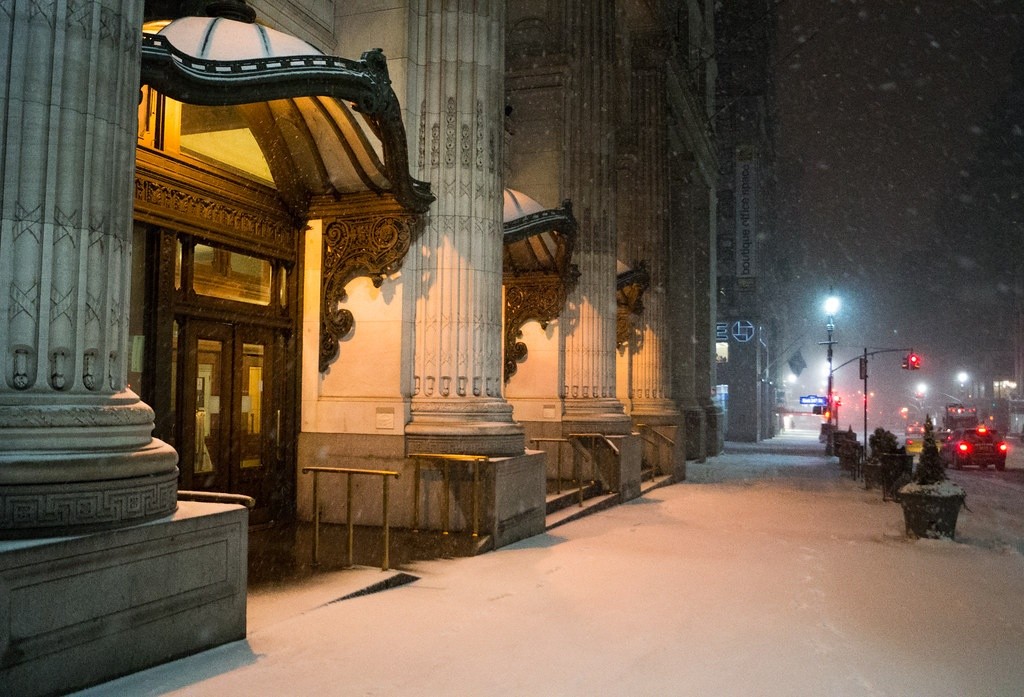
[906,402,1008,472]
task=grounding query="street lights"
[823,284,840,458]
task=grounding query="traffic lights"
[901,353,921,371]
